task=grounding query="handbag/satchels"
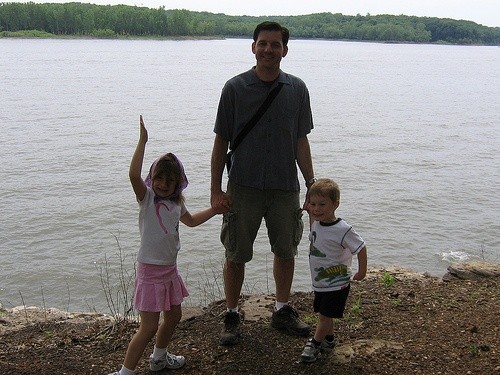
[224,148,235,177]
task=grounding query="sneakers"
[113,368,145,375]
[149,351,186,372]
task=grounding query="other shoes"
[271,303,311,337]
[322,338,336,349]
[218,304,246,347]
[300,341,322,363]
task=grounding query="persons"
[299,177,368,364]
[211,20,317,346]
[111,114,231,375]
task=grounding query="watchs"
[306,178,317,187]
